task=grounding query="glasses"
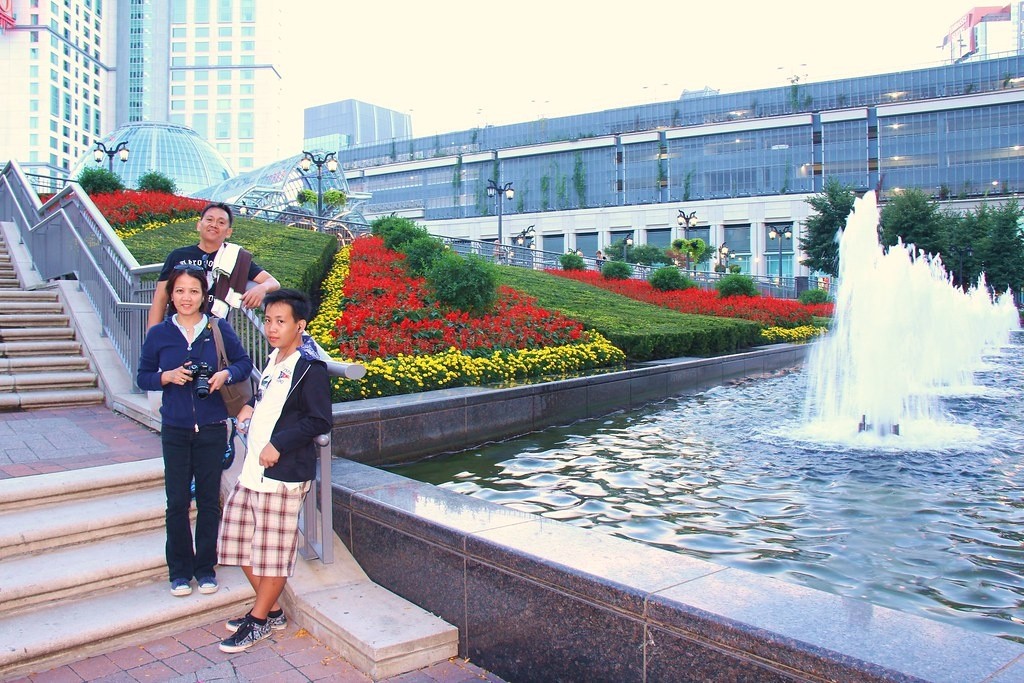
[170,264,204,279]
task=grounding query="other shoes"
[190,482,197,500]
[222,417,236,470]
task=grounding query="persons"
[137,263,253,596]
[146,204,281,500]
[218,288,332,653]
[595,250,607,273]
[492,240,506,265]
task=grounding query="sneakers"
[171,578,191,596]
[219,617,272,652]
[197,577,218,594]
[226,610,288,631]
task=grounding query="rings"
[180,378,186,383]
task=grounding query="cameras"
[185,361,214,401]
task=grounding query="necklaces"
[177,318,198,332]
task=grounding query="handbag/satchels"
[209,315,252,417]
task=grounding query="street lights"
[950,244,973,286]
[622,234,632,262]
[678,209,697,270]
[770,224,792,285]
[528,239,536,250]
[93,139,129,172]
[301,149,337,232]
[718,242,735,273]
[568,248,583,257]
[518,224,534,265]
[486,179,514,244]
[240,201,250,214]
